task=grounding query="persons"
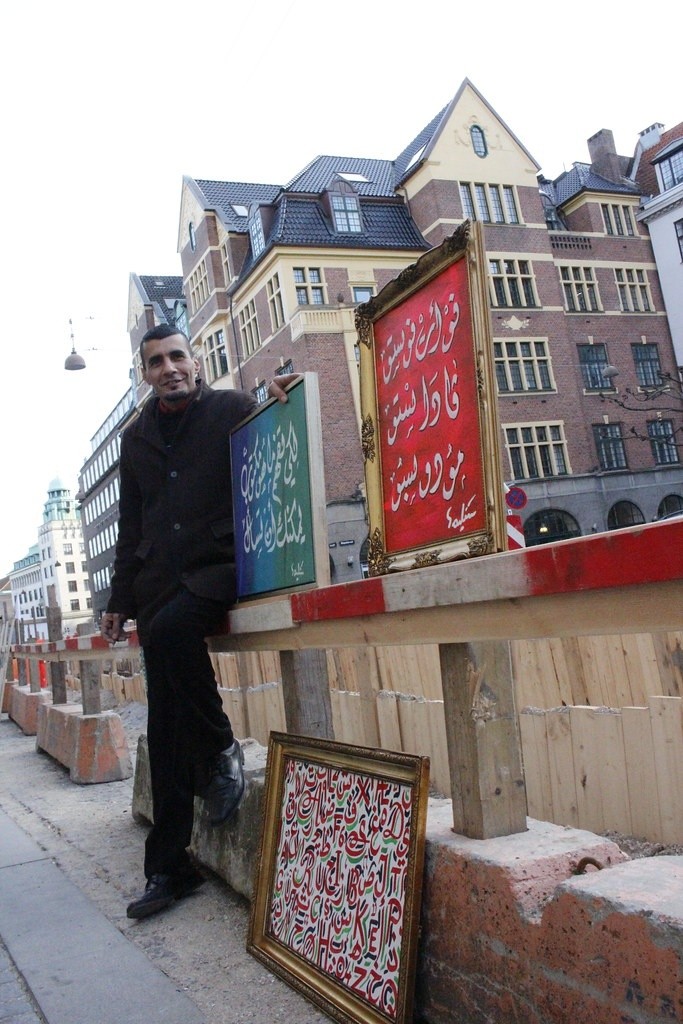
[100,325,302,919]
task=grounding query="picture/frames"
[353,219,509,577]
[227,371,331,603]
[245,730,430,1024]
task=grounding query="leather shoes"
[127,864,206,919]
[207,738,246,827]
